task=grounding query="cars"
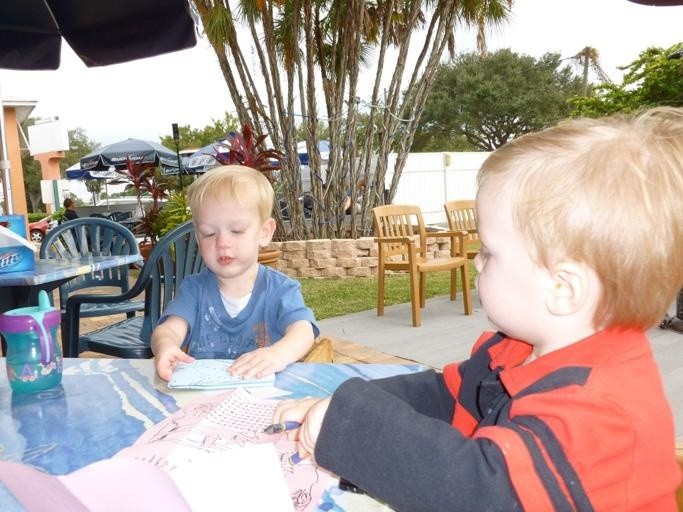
[28,215,52,242]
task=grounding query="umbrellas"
[0,0,196,69]
[66,138,345,216]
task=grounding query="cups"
[0,289,63,395]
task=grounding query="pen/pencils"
[289,451,311,465]
[265,421,302,434]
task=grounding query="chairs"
[373,199,479,328]
[38,215,206,360]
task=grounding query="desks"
[0,255,142,311]
[1,352,421,512]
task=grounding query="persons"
[151,165,320,379]
[63,199,80,220]
[276,106,682,512]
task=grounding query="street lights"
[172,123,183,189]
